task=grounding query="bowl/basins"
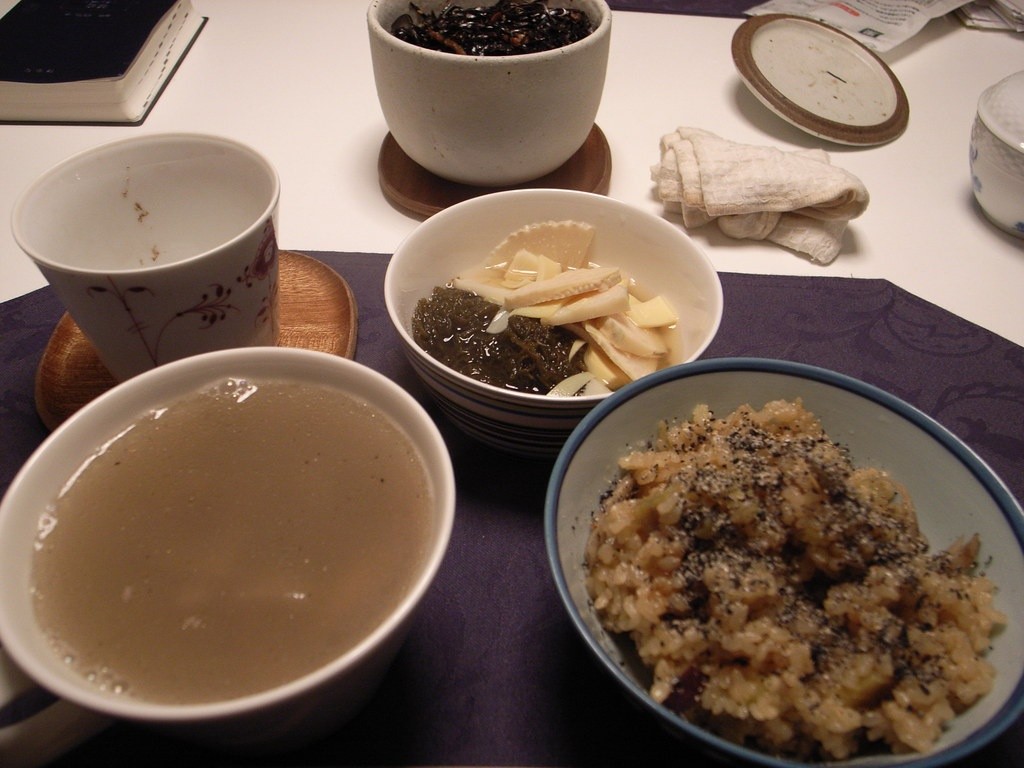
[11,133,281,383]
[969,68,1024,239]
[546,357,1024,768]
[0,346,457,751]
[367,0,612,187]
[384,188,725,461]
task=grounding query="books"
[0,0,209,127]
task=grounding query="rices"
[585,395,1006,759]
